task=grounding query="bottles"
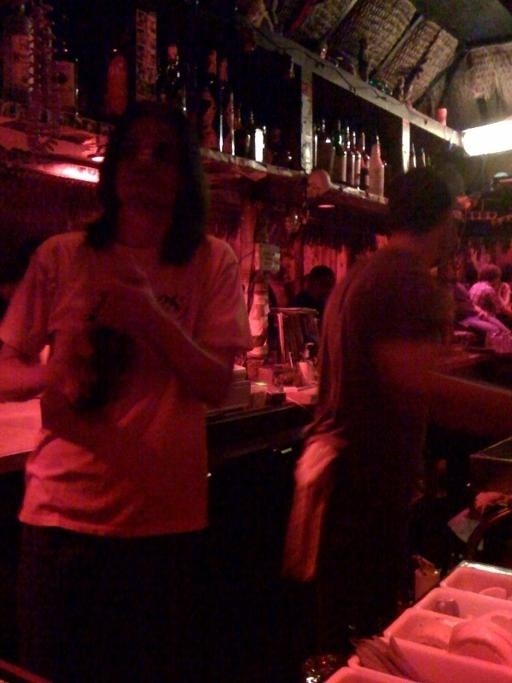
[312,117,392,198]
[0,0,272,165]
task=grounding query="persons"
[280,162,512,664]
[0,96,250,683]
[290,237,512,354]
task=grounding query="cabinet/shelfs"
[2,4,465,224]
